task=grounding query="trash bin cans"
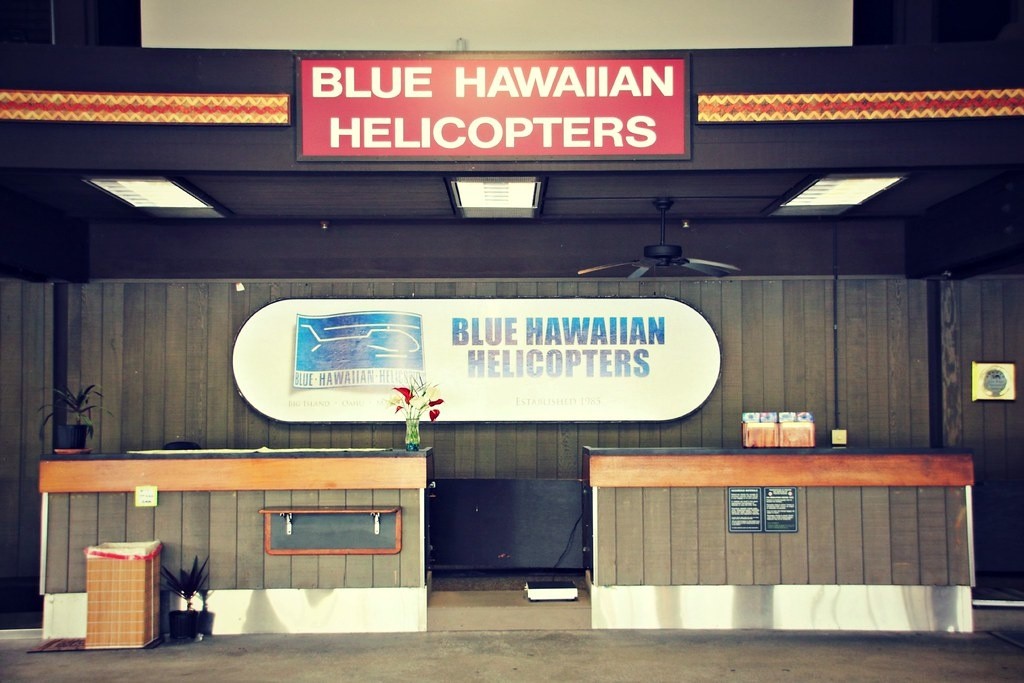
[82,541,163,650]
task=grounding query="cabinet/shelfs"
[583,446,977,634]
[40,447,434,639]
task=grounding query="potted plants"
[36,384,114,454]
[159,554,209,643]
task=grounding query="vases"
[405,418,420,451]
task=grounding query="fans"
[578,198,741,279]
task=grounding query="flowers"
[389,372,444,443]
